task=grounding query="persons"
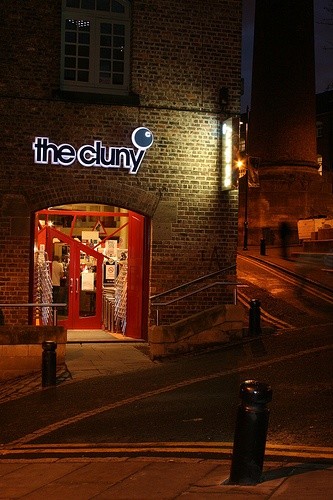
[87,266,97,313]
[52,256,64,311]
[277,216,294,258]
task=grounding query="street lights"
[237,154,249,250]
